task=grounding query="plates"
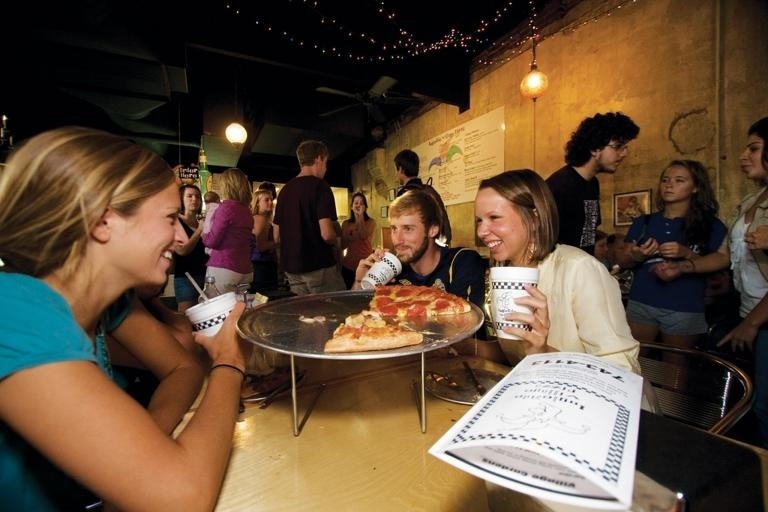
[421,354,513,406]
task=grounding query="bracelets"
[208,363,246,383]
[687,258,696,272]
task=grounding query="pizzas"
[370,284,471,317]
[325,309,423,352]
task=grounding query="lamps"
[517,19,549,104]
[222,99,248,152]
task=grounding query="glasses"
[605,143,622,150]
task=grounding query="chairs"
[630,340,757,435]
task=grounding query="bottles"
[197,277,223,304]
[197,150,212,196]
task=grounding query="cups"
[184,292,237,338]
[488,266,541,341]
[360,250,404,289]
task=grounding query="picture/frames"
[379,185,404,218]
[611,186,653,227]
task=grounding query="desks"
[173,351,688,510]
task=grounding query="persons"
[614,159,729,417]
[173,181,278,314]
[545,111,640,256]
[472,168,663,417]
[0,123,246,511]
[648,115,768,433]
[350,189,488,341]
[742,224,768,250]
[393,149,453,245]
[340,192,378,290]
[271,140,347,295]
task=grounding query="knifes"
[461,360,489,397]
